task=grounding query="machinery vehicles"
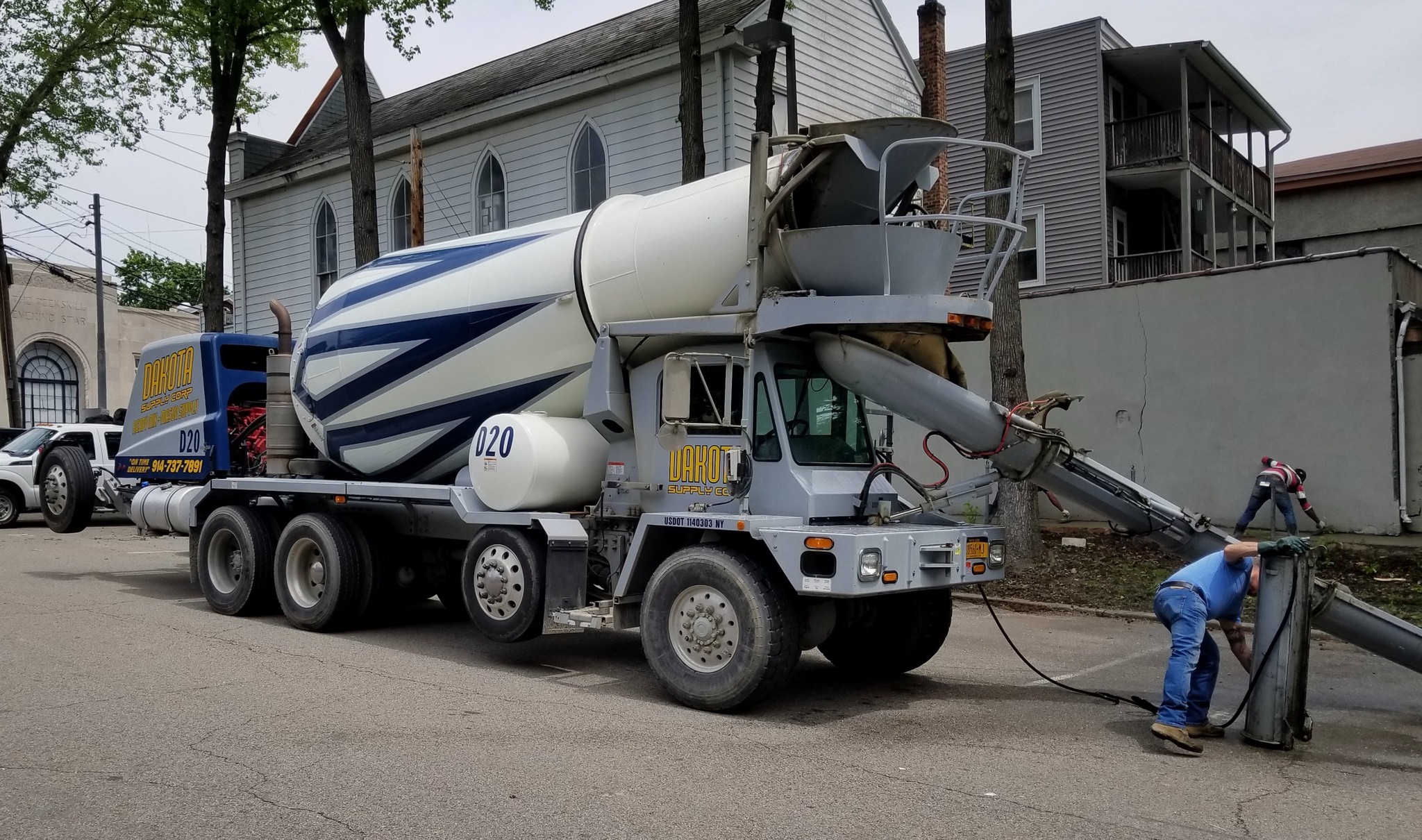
[31,118,1033,712]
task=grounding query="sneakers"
[1150,723,1204,753]
[1184,722,1225,737]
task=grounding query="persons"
[1151,535,1310,753]
[1233,457,1326,541]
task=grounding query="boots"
[1233,521,1248,540]
[1286,523,1299,537]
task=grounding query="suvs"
[0,423,123,529]
[1,427,27,449]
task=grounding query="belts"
[1259,474,1281,480]
[1157,581,1207,606]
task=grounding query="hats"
[1295,469,1306,485]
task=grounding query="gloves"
[1316,521,1326,529]
[1257,535,1311,560]
